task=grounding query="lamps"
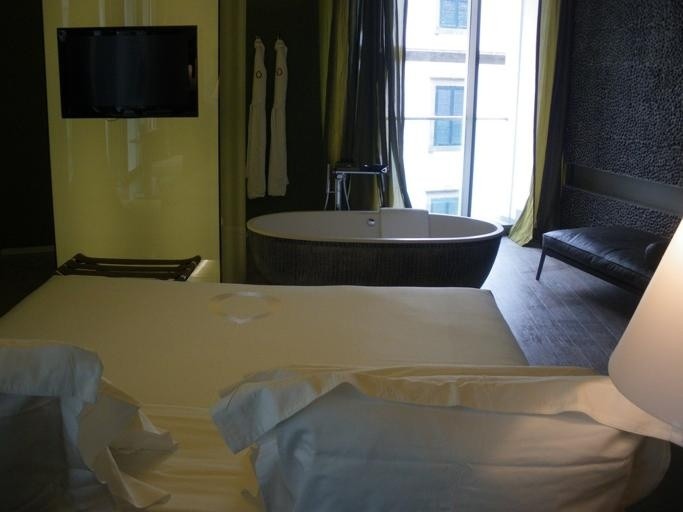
[606,215,683,435]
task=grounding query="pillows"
[80,370,178,462]
[642,236,670,270]
[248,361,604,380]
[0,337,171,512]
[208,375,673,511]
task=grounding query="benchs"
[536,225,670,297]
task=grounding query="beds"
[0,273,529,512]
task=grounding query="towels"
[267,39,289,196]
[244,38,266,200]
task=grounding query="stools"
[54,250,210,284]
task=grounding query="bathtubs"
[246,208,504,288]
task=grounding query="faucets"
[324,160,387,210]
